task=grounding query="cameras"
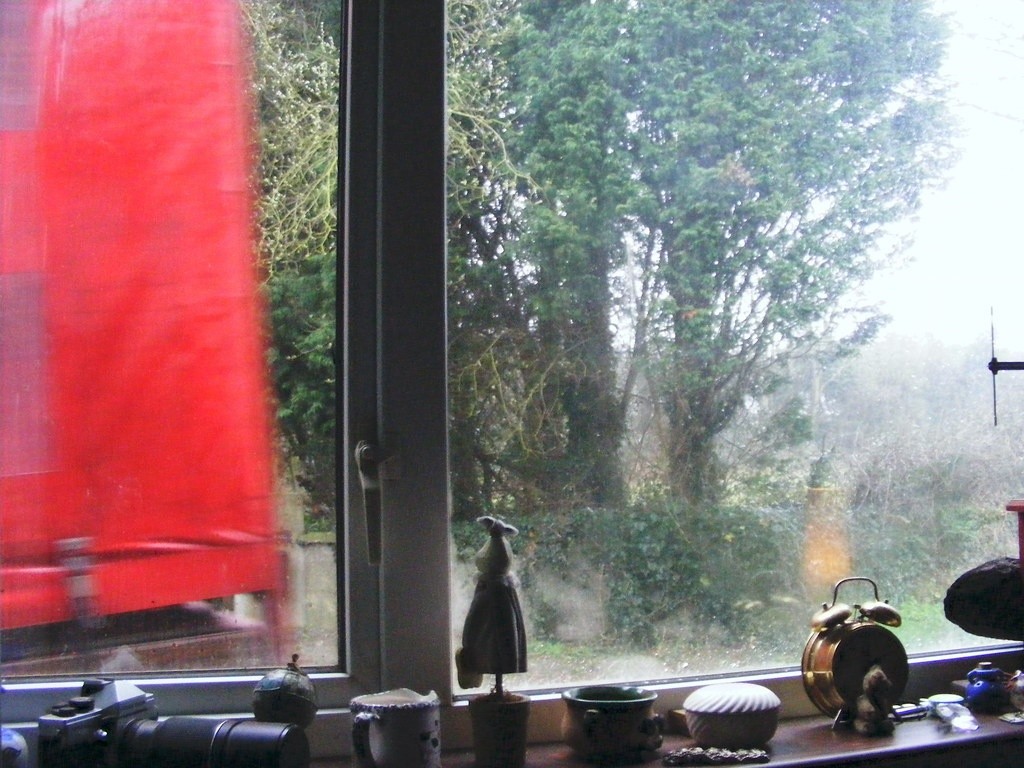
[37,679,311,768]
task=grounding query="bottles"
[965,668,1007,715]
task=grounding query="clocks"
[802,576,911,731]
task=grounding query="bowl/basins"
[560,686,663,764]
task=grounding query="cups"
[350,688,441,768]
